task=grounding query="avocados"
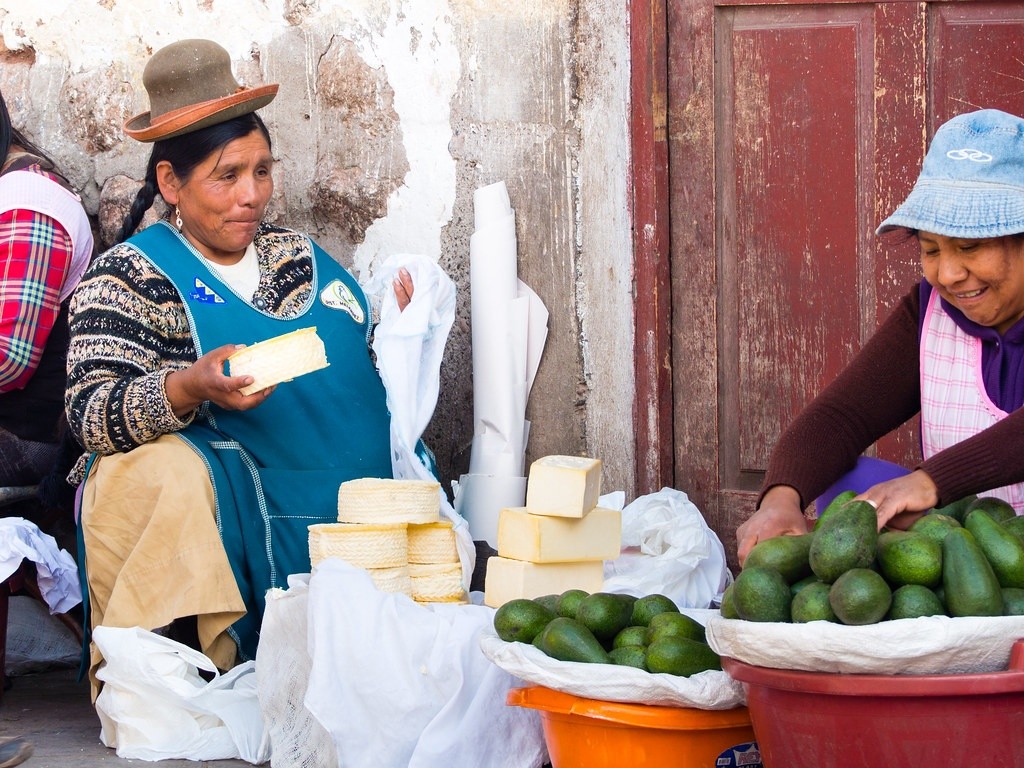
[718,489,1024,625]
[492,589,722,678]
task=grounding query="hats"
[121,38,282,145]
[875,109,1024,240]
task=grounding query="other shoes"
[0,736,34,768]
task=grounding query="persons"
[64,39,440,708]
[737,109,1024,568]
[0,91,95,485]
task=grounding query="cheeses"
[484,454,622,608]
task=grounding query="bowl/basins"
[721,653,1024,768]
[504,685,767,768]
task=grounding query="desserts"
[306,477,467,604]
[228,326,332,396]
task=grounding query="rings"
[866,500,878,510]
